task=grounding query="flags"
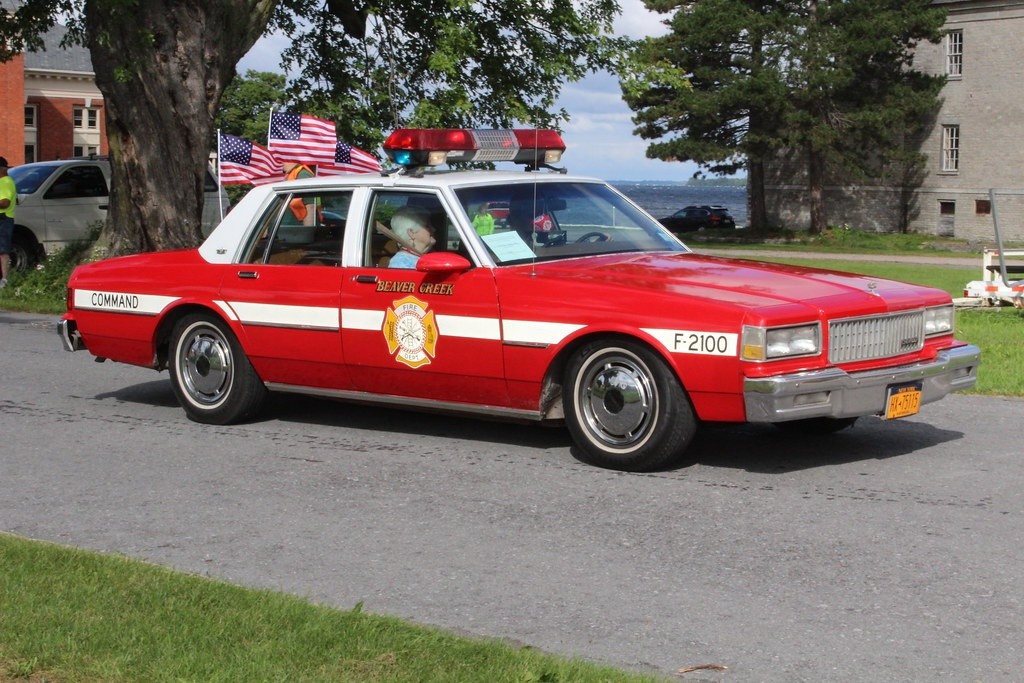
[216,110,383,198]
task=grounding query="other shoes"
[1,280,6,288]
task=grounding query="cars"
[54,124,982,475]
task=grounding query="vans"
[7,154,231,280]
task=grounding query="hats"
[0,156,8,167]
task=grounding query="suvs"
[657,205,738,232]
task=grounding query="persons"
[504,192,558,253]
[0,155,17,291]
[388,203,439,272]
[471,203,495,237]
